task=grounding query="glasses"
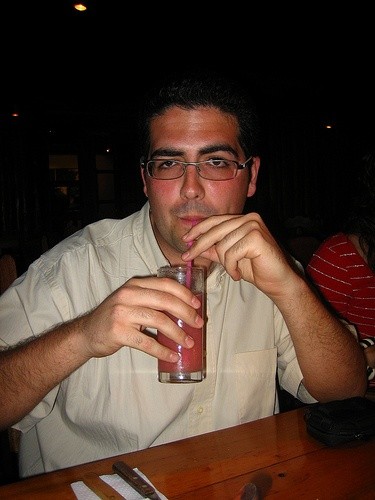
[141,158,251,181]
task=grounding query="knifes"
[113,461,161,500]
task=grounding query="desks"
[1,391,374,499]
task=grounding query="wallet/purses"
[304,396,375,447]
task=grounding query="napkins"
[70,466,167,500]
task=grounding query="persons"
[304,179,375,397]
[0,78,372,482]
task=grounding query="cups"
[157,267,204,383]
